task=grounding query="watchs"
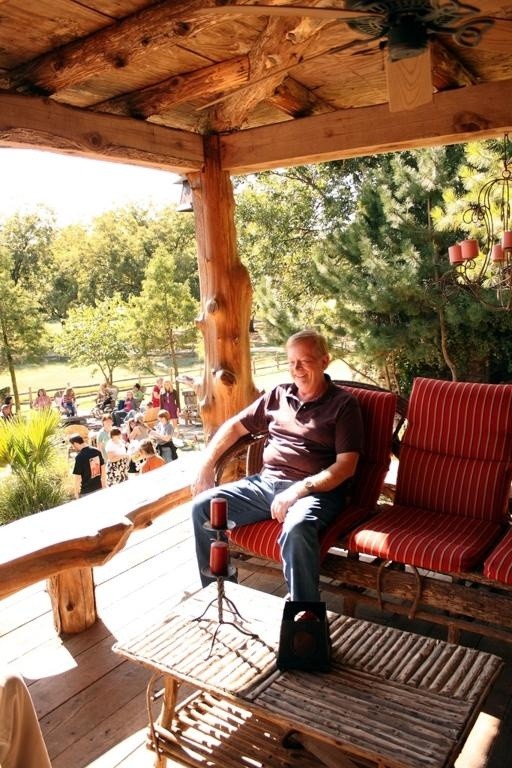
[302,480,315,495]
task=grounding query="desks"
[110,578,504,767]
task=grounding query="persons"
[192,329,365,600]
[0,376,180,500]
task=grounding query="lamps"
[424,134,512,313]
[190,498,254,661]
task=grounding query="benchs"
[214,374,512,644]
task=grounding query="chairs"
[53,391,78,417]
[65,424,89,458]
[139,407,160,430]
[93,385,119,414]
